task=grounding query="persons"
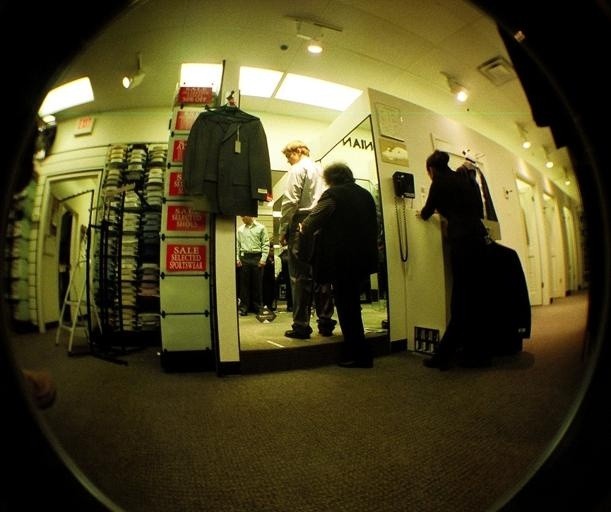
[369,182,389,328]
[299,164,379,368]
[278,140,337,339]
[236,215,293,315]
[419,149,495,368]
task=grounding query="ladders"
[55,225,102,357]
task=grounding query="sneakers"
[285,330,309,339]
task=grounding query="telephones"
[392,171,415,199]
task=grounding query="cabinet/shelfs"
[98,159,165,349]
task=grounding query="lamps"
[120,50,145,90]
[512,123,571,189]
[276,14,344,54]
[440,71,472,105]
